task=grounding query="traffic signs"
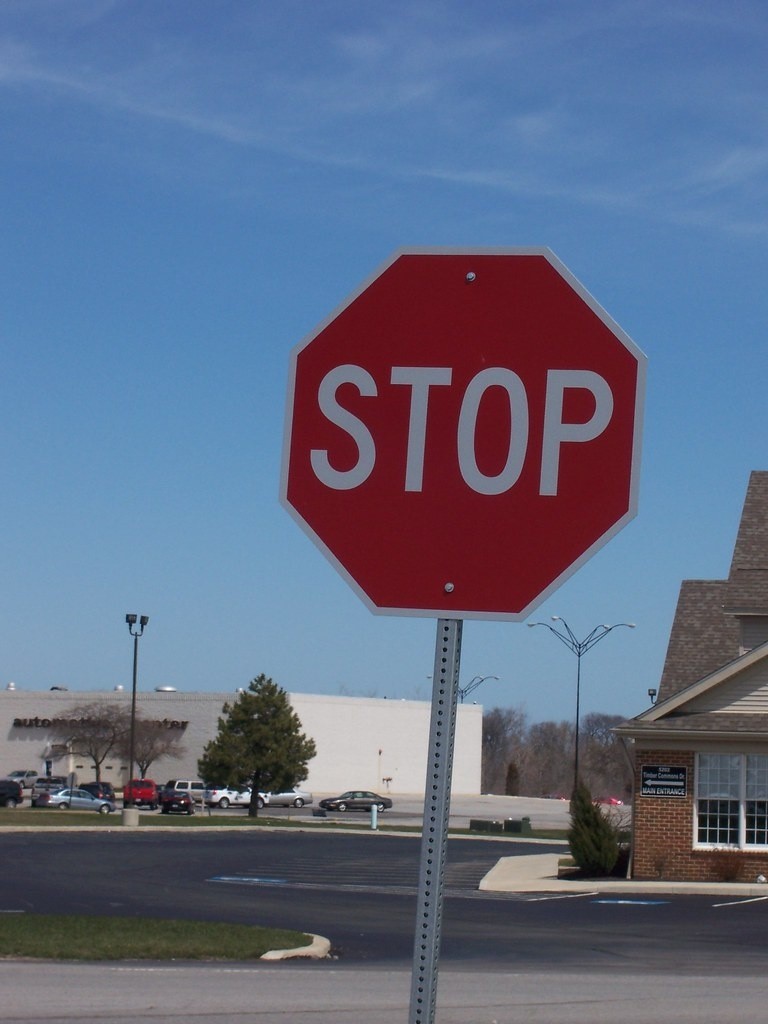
[640,765,688,798]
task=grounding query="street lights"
[523,616,638,790]
[426,673,500,703]
[125,613,150,780]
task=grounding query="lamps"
[648,688,657,703]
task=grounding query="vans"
[165,780,205,801]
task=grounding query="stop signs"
[279,245,646,622]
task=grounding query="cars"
[259,785,313,809]
[160,790,197,815]
[36,788,116,815]
[30,777,68,808]
[123,779,162,810]
[203,783,270,810]
[78,781,116,804]
[593,797,624,806]
[319,790,393,813]
[538,794,566,800]
[6,769,39,789]
[0,780,23,808]
